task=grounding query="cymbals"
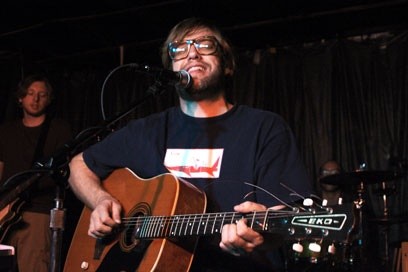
[320,172,370,186]
[371,188,394,195]
[369,216,408,225]
[366,171,398,183]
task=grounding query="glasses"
[320,169,337,175]
[168,38,223,60]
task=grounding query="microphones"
[136,63,192,89]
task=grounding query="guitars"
[0,159,54,243]
[63,167,363,272]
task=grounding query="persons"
[67,16,313,272]
[0,74,73,272]
[315,160,379,272]
